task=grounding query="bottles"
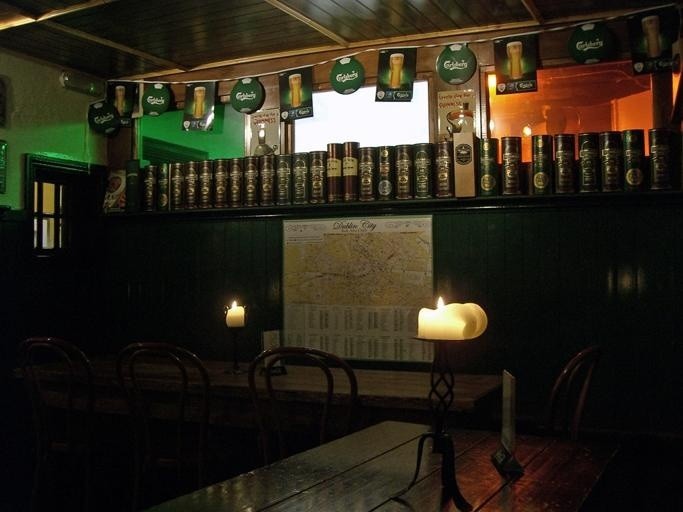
[253,129,274,156]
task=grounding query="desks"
[1,358,506,439]
[148,418,681,509]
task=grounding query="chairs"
[248,345,358,431]
[116,341,210,432]
[543,346,606,433]
[19,336,97,422]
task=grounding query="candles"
[418,295,488,338]
[225,300,246,327]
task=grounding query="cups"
[505,41,524,81]
[285,71,304,109]
[387,53,405,90]
[641,13,659,62]
[191,84,207,118]
[113,83,128,114]
